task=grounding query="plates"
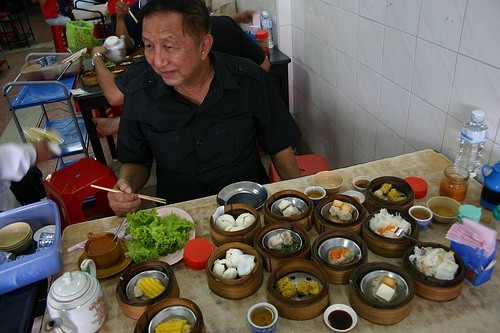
[156,207,195,265]
[77,239,132,278]
[22,126,64,145]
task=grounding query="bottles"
[439,165,470,204]
[455,109,488,178]
[489,205,500,241]
[457,205,482,225]
[256,11,274,59]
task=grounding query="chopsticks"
[121,0,138,24]
[90,184,166,204]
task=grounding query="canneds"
[438,165,470,202]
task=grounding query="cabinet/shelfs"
[2,52,94,173]
[0,0,35,50]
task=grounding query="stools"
[269,154,331,183]
[50,26,68,53]
[43,157,118,231]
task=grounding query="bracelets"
[92,53,106,66]
[209,13,213,16]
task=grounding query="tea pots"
[102,34,126,61]
[43,258,107,333]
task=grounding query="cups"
[408,205,433,231]
[84,232,121,268]
[247,302,279,333]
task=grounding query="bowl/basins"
[360,269,408,306]
[125,270,169,300]
[216,170,374,267]
[426,196,462,223]
[148,305,196,333]
[0,221,55,253]
[323,304,358,333]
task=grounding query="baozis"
[216,213,256,231]
[213,248,256,279]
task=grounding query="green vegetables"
[82,54,116,67]
[281,243,299,253]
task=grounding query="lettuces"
[123,209,196,263]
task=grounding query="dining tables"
[40,149,500,333]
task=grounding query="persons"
[40,0,71,25]
[0,141,51,214]
[107,0,299,215]
[115,0,256,56]
[92,0,270,136]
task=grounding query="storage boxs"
[0,199,62,295]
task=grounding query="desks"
[73,46,291,167]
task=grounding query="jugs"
[480,161,500,211]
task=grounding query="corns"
[137,276,165,299]
[154,319,191,333]
